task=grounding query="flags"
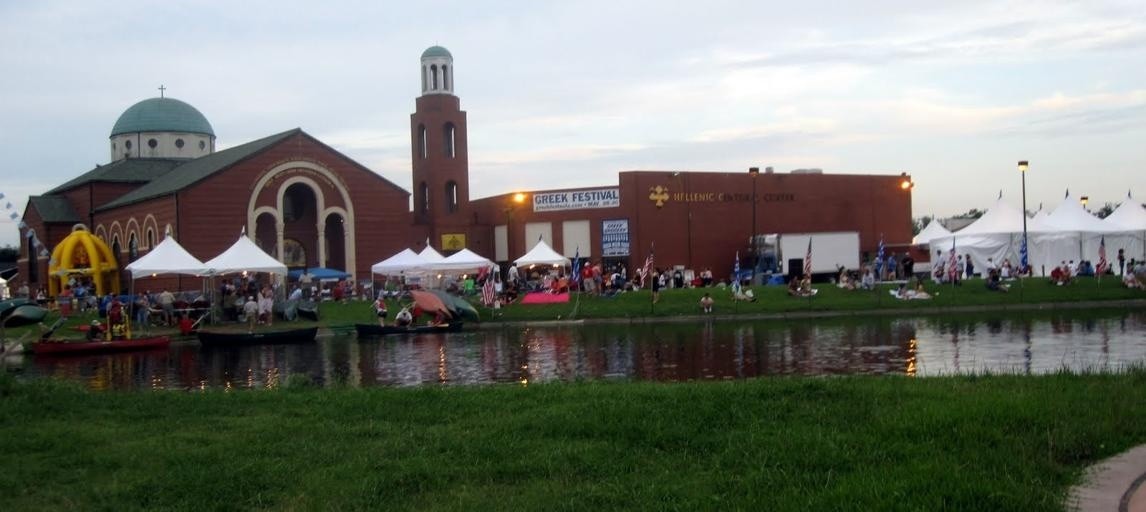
[734,254,742,290]
[481,268,495,306]
[802,236,814,281]
[876,239,888,273]
[640,241,655,282]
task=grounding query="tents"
[283,268,353,301]
[910,185,1146,281]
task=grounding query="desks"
[151,307,210,320]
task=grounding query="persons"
[984,258,1013,292]
[506,263,523,303]
[549,277,560,295]
[332,280,443,327]
[298,268,315,299]
[219,278,274,334]
[1054,249,1146,291]
[46,295,59,313]
[582,262,627,297]
[652,267,756,312]
[13,278,31,298]
[840,250,974,300]
[35,282,47,306]
[59,273,176,342]
[787,273,811,297]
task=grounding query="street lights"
[505,194,526,261]
[749,167,759,279]
[1018,161,1029,231]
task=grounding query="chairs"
[107,301,131,341]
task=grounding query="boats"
[197,326,320,344]
[22,336,171,356]
[355,323,450,334]
